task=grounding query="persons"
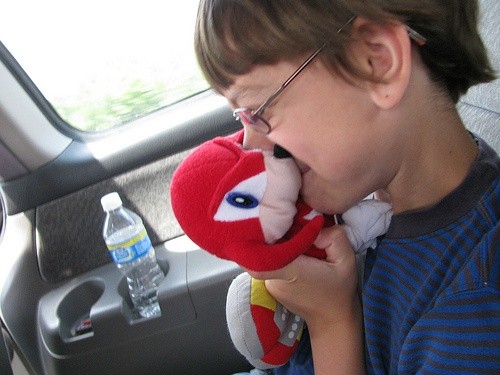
[194,0,500,375]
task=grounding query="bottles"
[100,191,165,319]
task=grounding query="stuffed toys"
[170,129,393,369]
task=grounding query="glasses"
[231,11,434,134]
